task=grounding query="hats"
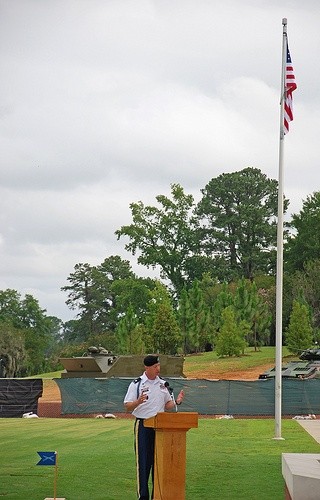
[144,356,160,366]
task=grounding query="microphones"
[164,382,173,397]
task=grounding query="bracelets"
[175,398,183,406]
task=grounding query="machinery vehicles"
[258,346,320,380]
[58,346,187,378]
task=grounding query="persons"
[124,353,186,500]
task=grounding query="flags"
[279,28,298,137]
